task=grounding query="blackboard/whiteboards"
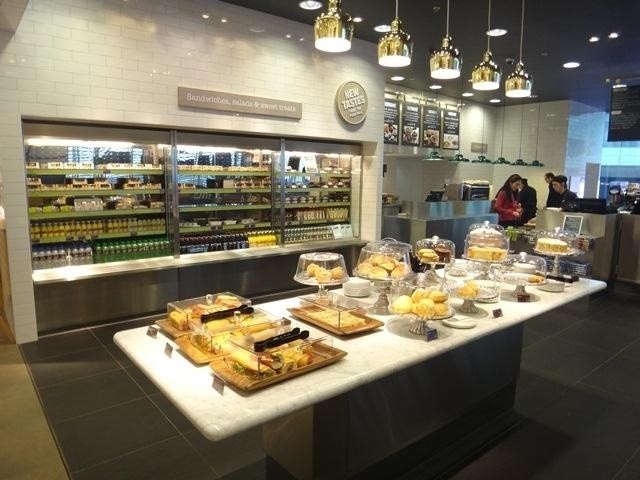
[562,215,583,234]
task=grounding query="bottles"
[179,229,277,256]
[31,238,171,270]
[284,225,353,244]
[28,215,165,241]
[285,207,349,224]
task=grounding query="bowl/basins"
[430,135,437,144]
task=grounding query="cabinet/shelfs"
[23,117,174,270]
[174,128,281,259]
[280,135,364,245]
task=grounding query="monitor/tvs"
[576,199,606,212]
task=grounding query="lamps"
[427,0,463,82]
[375,0,415,67]
[415,62,545,168]
[311,0,357,54]
[469,0,502,92]
[504,0,534,100]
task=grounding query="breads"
[468,246,506,261]
[458,281,479,298]
[359,247,452,278]
[307,263,345,281]
[392,288,449,317]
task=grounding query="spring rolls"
[227,332,313,379]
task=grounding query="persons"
[494,174,524,231]
[518,178,538,223]
[544,172,562,208]
[551,175,577,208]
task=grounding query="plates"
[442,314,476,329]
[343,277,373,298]
[540,283,566,294]
[410,130,419,143]
[513,263,535,274]
[444,135,458,148]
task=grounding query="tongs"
[254,328,311,351]
[200,304,254,324]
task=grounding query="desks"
[112,258,608,480]
